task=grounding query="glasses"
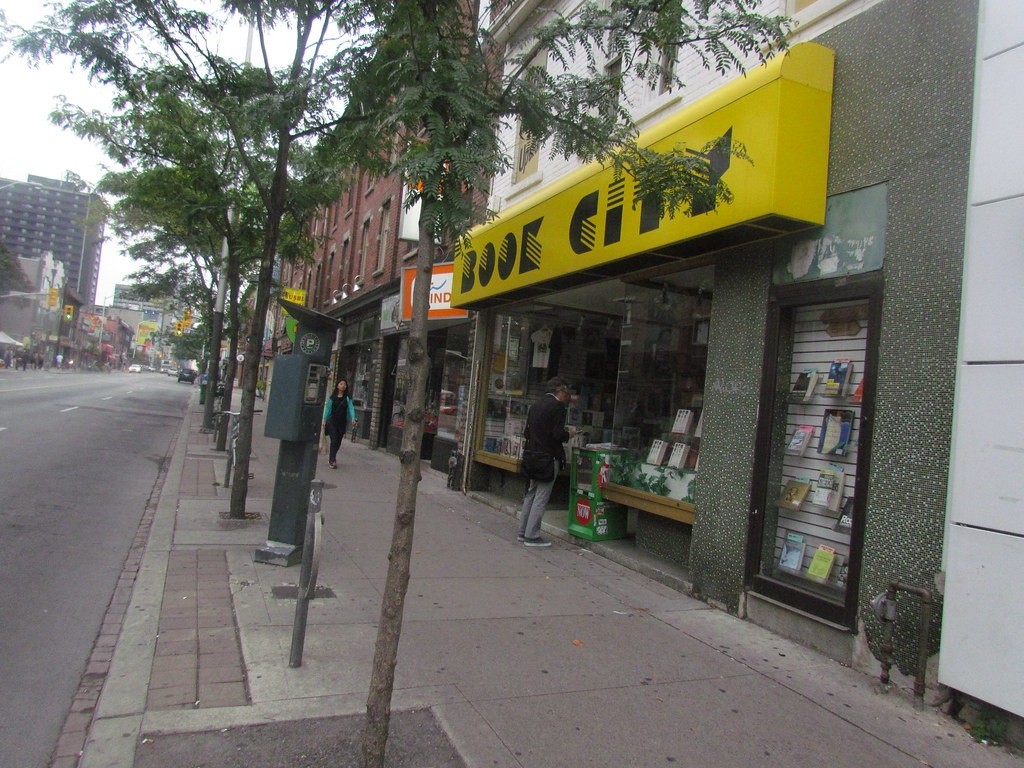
[560,387,570,396]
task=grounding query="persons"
[322,379,356,469]
[4,347,45,371]
[516,375,577,547]
[56,353,63,369]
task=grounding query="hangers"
[532,317,574,338]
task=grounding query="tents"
[0,331,23,350]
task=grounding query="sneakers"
[328,459,337,469]
[517,533,524,541]
[523,537,552,547]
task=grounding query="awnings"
[450,42,834,317]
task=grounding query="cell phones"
[575,430,584,434]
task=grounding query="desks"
[475,448,571,483]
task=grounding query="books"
[777,362,864,587]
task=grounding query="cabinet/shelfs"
[601,392,700,530]
[772,390,864,599]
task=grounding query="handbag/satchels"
[319,424,330,456]
[521,451,555,482]
